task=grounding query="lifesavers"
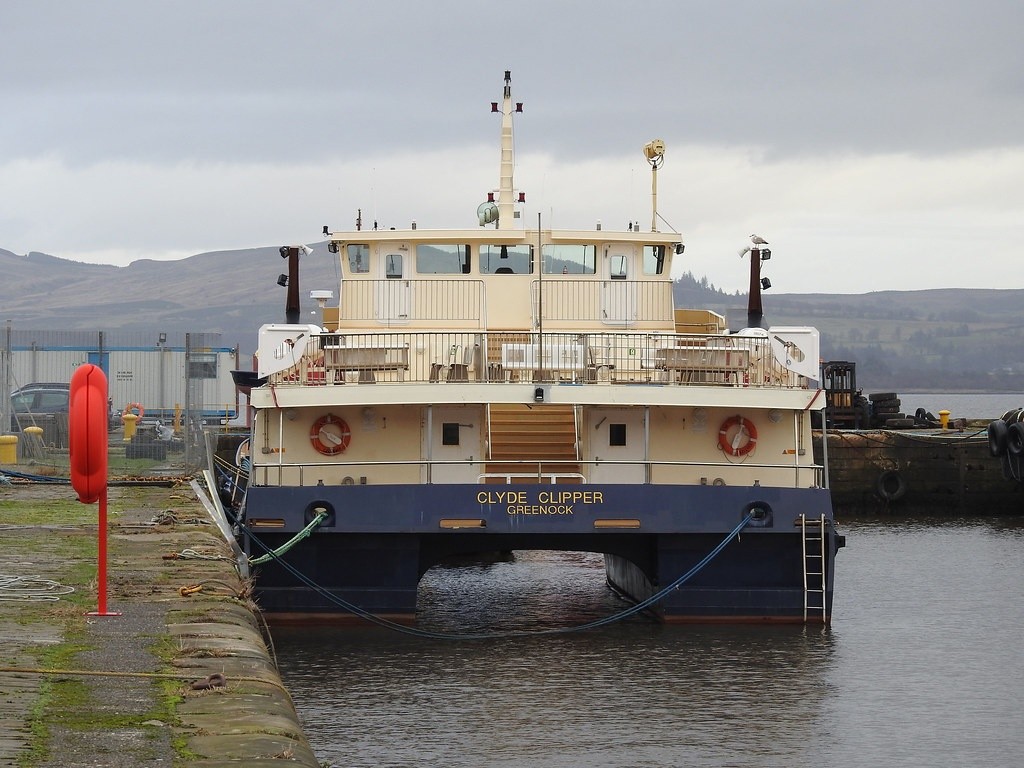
[121,402,144,423]
[724,371,749,388]
[718,416,757,456]
[310,415,351,456]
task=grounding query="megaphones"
[299,243,313,257]
[477,202,498,224]
[737,246,750,258]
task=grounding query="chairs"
[430,344,461,383]
[447,343,480,383]
[588,346,614,370]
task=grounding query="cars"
[10,382,70,447]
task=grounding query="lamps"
[761,277,771,291]
[415,342,427,355]
[676,244,685,255]
[279,246,289,259]
[277,274,289,287]
[761,249,771,260]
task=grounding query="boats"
[231,66,847,622]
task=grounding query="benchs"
[656,347,750,387]
[502,344,588,384]
[324,343,409,382]
